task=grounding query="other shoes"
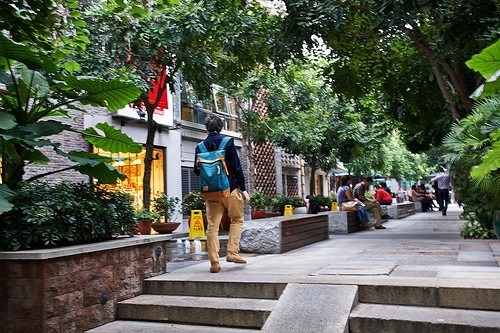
[227,256,246,264]
[381,214,394,220]
[210,262,220,272]
[375,225,386,229]
[367,219,377,227]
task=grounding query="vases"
[221,213,231,232]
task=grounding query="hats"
[364,176,374,185]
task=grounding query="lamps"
[137,110,145,120]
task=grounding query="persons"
[352,176,393,229]
[305,195,310,208]
[411,182,437,211]
[337,175,377,228]
[193,116,250,273]
[374,185,392,205]
[331,189,334,195]
[398,188,407,203]
[432,168,451,215]
[380,182,390,193]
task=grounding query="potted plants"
[136,206,157,235]
[180,191,207,230]
[152,190,182,234]
[250,190,331,219]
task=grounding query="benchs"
[241,214,330,254]
[326,210,363,234]
[387,201,415,219]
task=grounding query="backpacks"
[195,136,233,202]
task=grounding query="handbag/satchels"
[342,201,360,211]
